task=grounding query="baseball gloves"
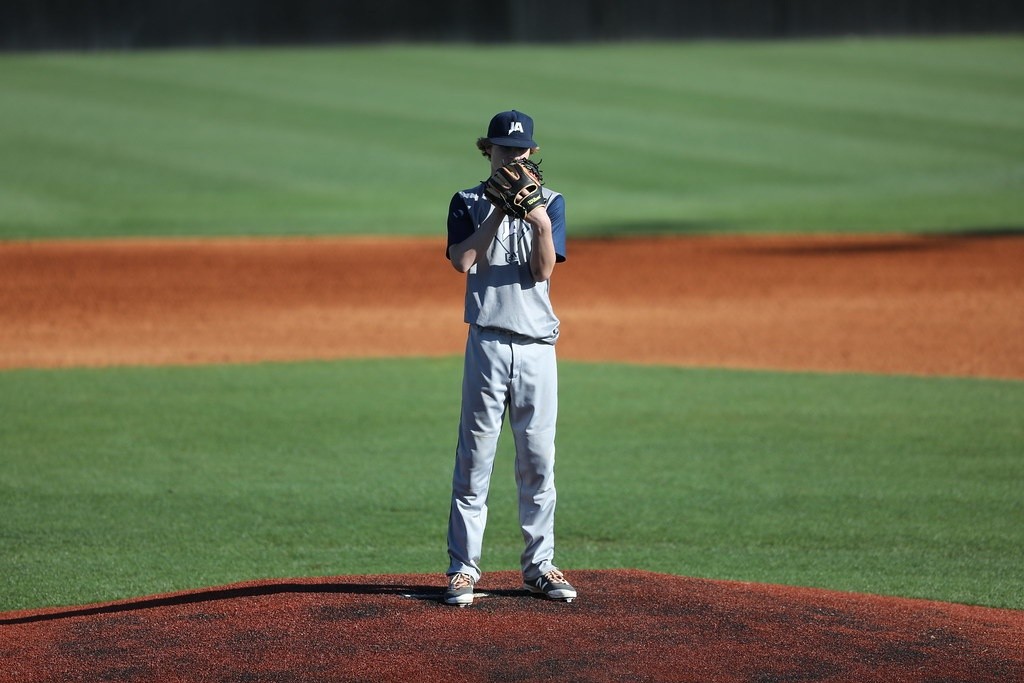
[479,157,548,221]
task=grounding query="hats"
[487,110,538,148]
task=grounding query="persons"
[444,107,579,607]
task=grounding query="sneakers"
[523,569,577,602]
[444,573,474,608]
